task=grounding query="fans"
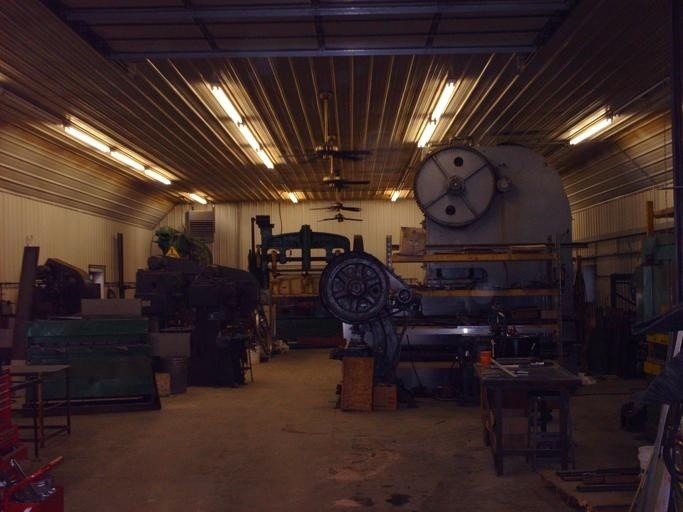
[279,90,372,223]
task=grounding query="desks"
[4,364,72,447]
[472,362,583,479]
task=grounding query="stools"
[524,390,576,471]
